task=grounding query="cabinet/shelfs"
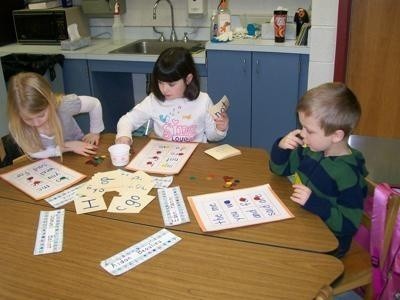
[61,49,309,149]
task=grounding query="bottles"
[211,8,232,42]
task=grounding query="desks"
[0,132,344,300]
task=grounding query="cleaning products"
[112,2,124,44]
[217,0,232,43]
[210,10,219,43]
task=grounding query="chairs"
[332,177,400,300]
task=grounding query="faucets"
[153,0,174,28]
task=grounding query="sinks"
[108,39,208,56]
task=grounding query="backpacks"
[350,182,400,300]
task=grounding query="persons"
[112,45,231,148]
[268,81,369,287]
[7,72,107,157]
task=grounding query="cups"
[108,144,130,167]
[273,9,288,42]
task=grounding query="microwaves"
[12,5,92,46]
